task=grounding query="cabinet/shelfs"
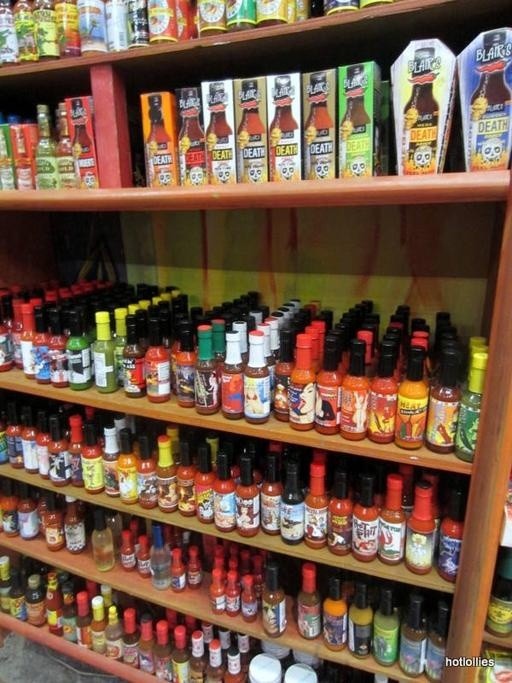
[0,0,512,683]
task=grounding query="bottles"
[340,65,371,178]
[205,82,234,183]
[70,99,97,189]
[400,41,439,176]
[0,272,511,683]
[32,99,77,192]
[303,71,335,182]
[466,32,512,171]
[177,88,207,187]
[236,79,267,181]
[0,127,34,193]
[146,94,173,187]
[268,75,300,183]
[0,0,395,66]
